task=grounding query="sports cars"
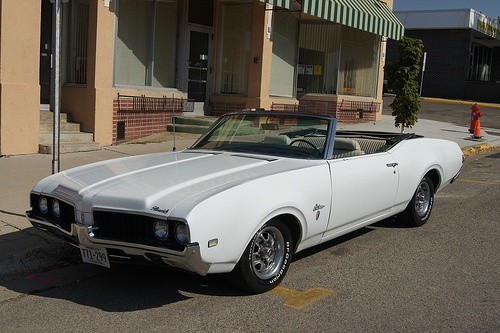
[25,110,465,294]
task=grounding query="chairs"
[335,138,361,154]
[264,135,291,145]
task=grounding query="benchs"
[299,137,386,155]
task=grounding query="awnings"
[261,0,294,10]
[301,0,404,42]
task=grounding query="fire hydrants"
[468,103,483,133]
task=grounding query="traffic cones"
[472,118,485,141]
